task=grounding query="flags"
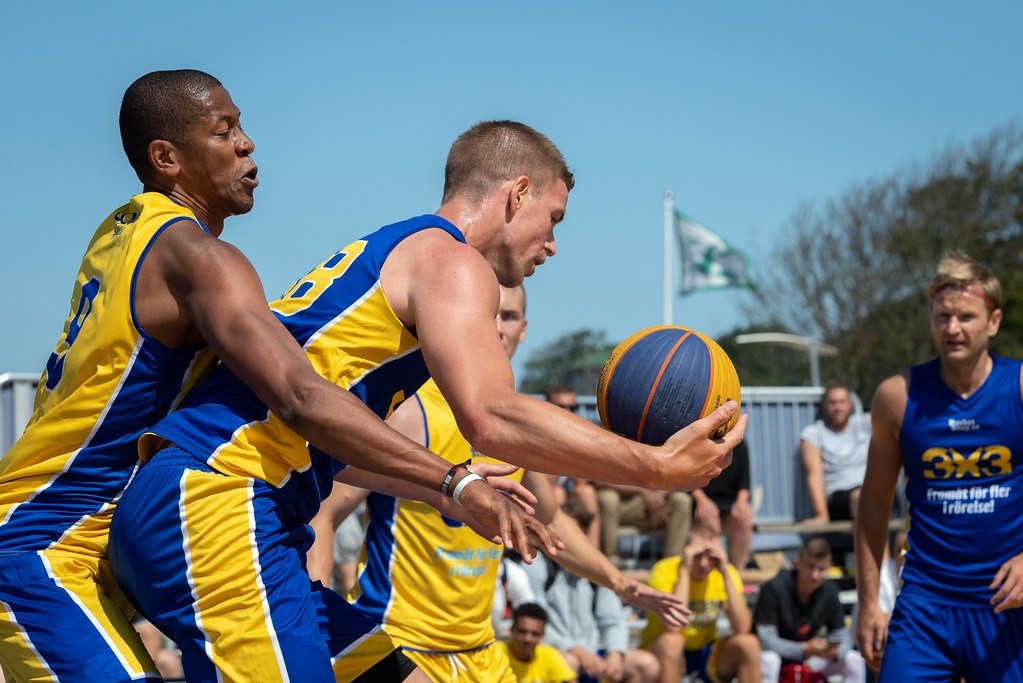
[676,210,764,302]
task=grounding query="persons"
[133,283,908,683]
[0,71,567,683]
[110,122,749,683]
[857,262,1023,683]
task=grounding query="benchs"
[618,519,906,538]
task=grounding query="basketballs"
[597,325,741,447]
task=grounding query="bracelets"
[442,459,472,497]
[453,474,485,507]
